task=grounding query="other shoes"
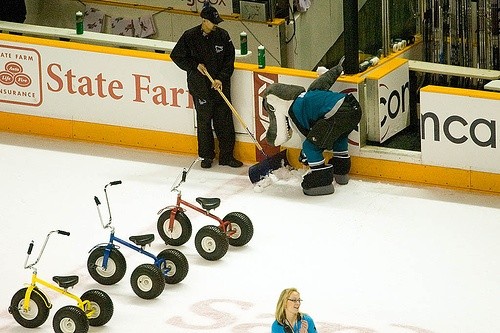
[218,156,244,168]
[201,158,213,168]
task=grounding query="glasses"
[288,297,303,303]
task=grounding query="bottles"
[258,45,265,69]
[240,31,247,55]
[76,10,83,35]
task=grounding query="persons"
[271,288,318,333]
[169,6,244,168]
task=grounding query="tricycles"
[86,179,190,299]
[7,230,114,333]
[156,158,254,260]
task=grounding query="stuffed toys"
[263,55,362,196]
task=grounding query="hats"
[200,7,224,24]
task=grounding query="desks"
[0,20,252,58]
[408,58,500,91]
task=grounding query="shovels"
[203,68,297,188]
